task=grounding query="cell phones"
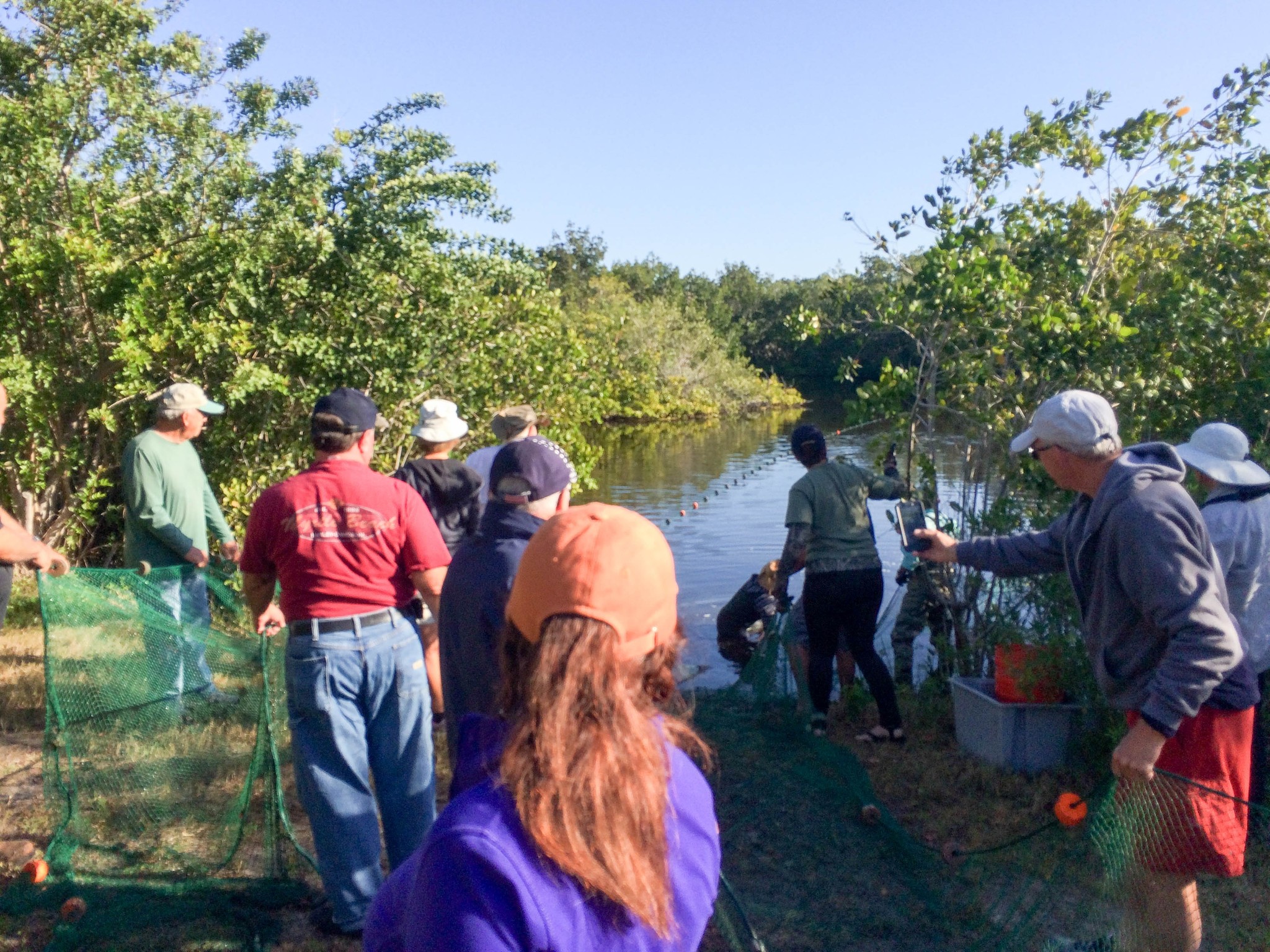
[895,500,932,552]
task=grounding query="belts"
[289,607,407,636]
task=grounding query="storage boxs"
[947,676,1084,778]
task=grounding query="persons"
[898,390,1259,952]
[716,426,983,745]
[438,436,577,801]
[0,381,69,625]
[242,388,453,939]
[396,399,538,729]
[121,383,241,726]
[359,502,719,952]
[1175,421,1270,867]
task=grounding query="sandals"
[854,724,906,744]
[808,722,827,739]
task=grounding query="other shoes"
[180,713,194,724]
[431,717,447,733]
[1074,935,1115,952]
[206,690,241,706]
[309,906,365,939]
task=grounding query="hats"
[489,437,577,505]
[506,501,677,661]
[1011,390,1117,452]
[791,425,824,453]
[311,388,389,433]
[490,405,548,439]
[411,399,468,443]
[159,383,224,414]
[913,477,932,491]
[1175,423,1270,486]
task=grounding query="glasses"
[1032,444,1061,460]
